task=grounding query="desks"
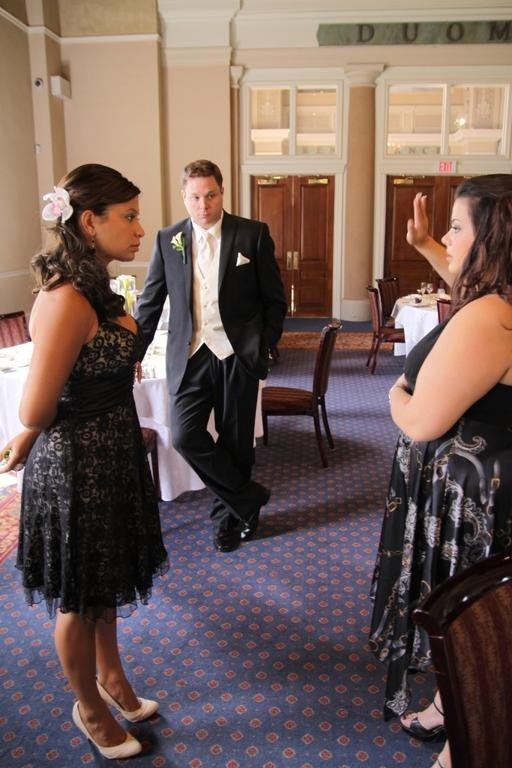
[0,329,265,500]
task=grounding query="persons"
[405,191,455,289]
[366,175,512,767]
[134,159,289,552]
[0,163,158,761]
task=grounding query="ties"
[200,233,213,276]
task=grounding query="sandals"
[400,701,446,742]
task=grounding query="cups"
[438,288,445,299]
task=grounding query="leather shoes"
[214,486,270,552]
[72,681,159,760]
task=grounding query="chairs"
[262,321,339,467]
[374,277,399,354]
[410,554,512,768]
[141,428,161,501]
[437,300,454,324]
[367,286,405,374]
[0,311,29,347]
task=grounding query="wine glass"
[421,282,434,303]
